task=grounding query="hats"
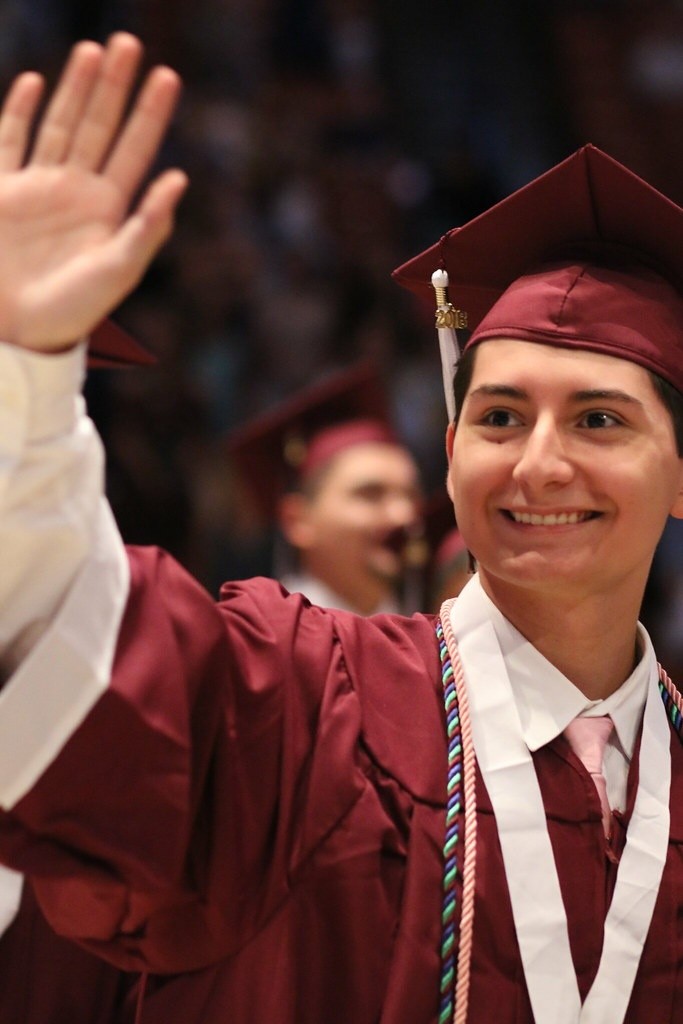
[227,365,403,534]
[390,142,683,575]
[87,318,161,373]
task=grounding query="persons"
[1,32,683,1023]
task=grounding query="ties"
[561,716,613,847]
